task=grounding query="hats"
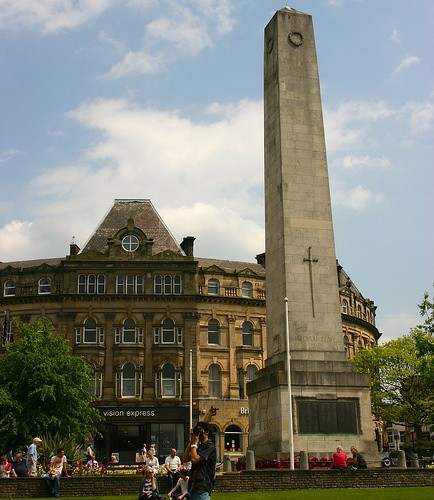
[33,437,42,442]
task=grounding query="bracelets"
[190,444,198,449]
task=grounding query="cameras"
[193,425,203,437]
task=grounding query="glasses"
[148,471,154,474]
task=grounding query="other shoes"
[50,489,59,498]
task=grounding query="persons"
[165,448,181,494]
[350,446,367,469]
[26,437,42,477]
[331,445,348,469]
[0,449,28,478]
[142,443,156,458]
[168,468,190,500]
[85,443,99,470]
[146,450,160,469]
[182,421,216,500]
[138,466,167,500]
[45,448,71,498]
